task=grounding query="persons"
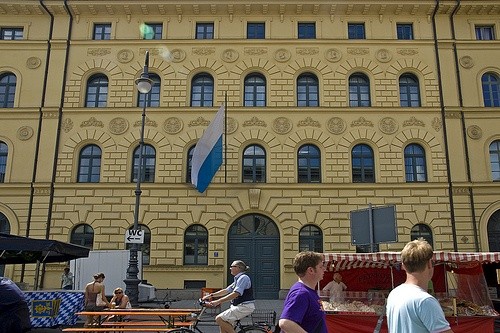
[84,273,110,328]
[386,237,460,333]
[323,273,348,304]
[61,267,73,290]
[278,251,327,333]
[105,288,132,333]
[197,260,255,333]
[0,277,32,333]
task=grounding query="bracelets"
[210,294,213,298]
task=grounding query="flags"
[191,103,225,193]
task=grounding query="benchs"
[62,318,201,333]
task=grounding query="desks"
[74,309,202,333]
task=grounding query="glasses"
[230,266,237,268]
[115,292,122,294]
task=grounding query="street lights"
[124,50,154,307]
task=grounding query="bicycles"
[166,299,273,333]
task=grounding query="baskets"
[251,309,276,326]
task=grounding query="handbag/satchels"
[96,284,106,306]
